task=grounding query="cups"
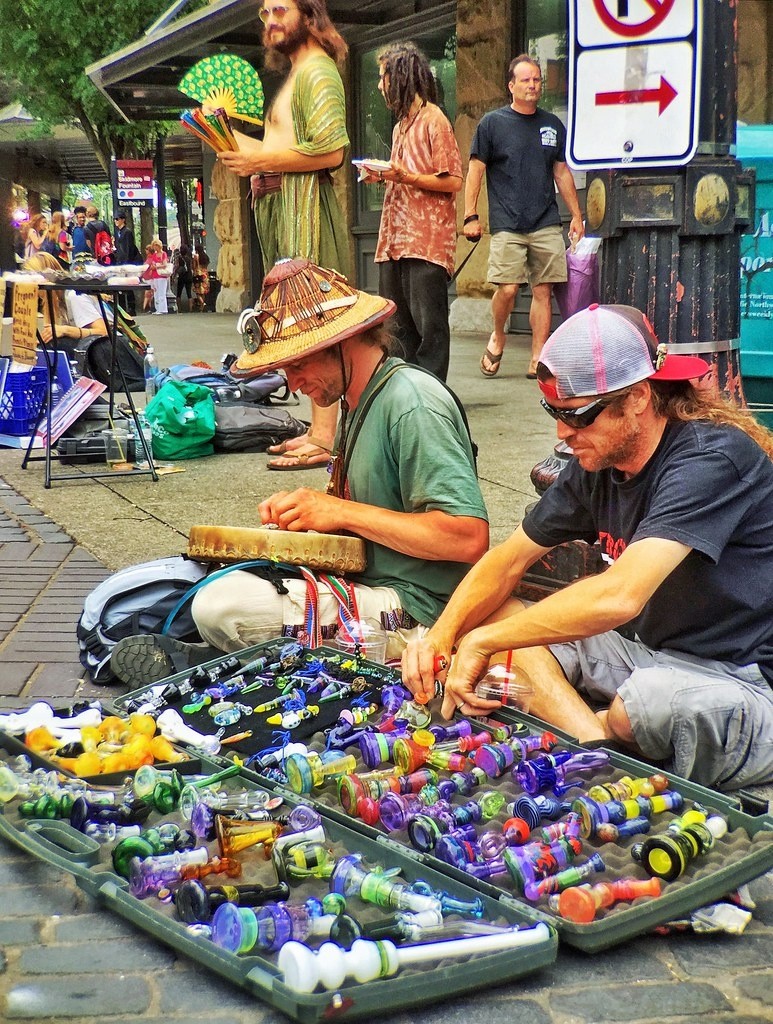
[475,662,535,715]
[334,616,390,665]
[102,428,130,469]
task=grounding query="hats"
[227,256,397,378]
[536,303,709,399]
[113,212,126,218]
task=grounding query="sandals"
[266,429,337,472]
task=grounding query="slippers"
[479,331,506,376]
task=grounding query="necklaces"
[392,100,422,159]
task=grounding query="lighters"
[433,654,447,675]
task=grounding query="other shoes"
[152,311,167,315]
[200,303,207,312]
[112,634,218,688]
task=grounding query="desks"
[6,280,159,489]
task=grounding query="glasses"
[539,394,621,430]
[115,218,120,221]
[258,6,298,22]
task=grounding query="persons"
[21,205,210,315]
[463,56,583,373]
[112,260,491,690]
[359,38,463,384]
[403,302,773,796]
[215,0,354,471]
[22,251,150,394]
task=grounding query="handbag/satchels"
[156,263,174,276]
[144,353,315,462]
[176,262,189,279]
[77,555,206,685]
[554,234,600,321]
[192,275,203,283]
[84,334,148,392]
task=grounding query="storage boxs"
[56,436,136,465]
[37,312,44,344]
[0,367,49,437]
[0,636,773,1024]
[1,317,12,357]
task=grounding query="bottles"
[134,411,153,464]
[144,347,158,403]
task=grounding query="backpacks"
[86,220,116,266]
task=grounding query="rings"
[455,701,465,711]
[378,171,382,177]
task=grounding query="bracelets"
[395,172,407,185]
[78,326,84,340]
[89,328,92,336]
